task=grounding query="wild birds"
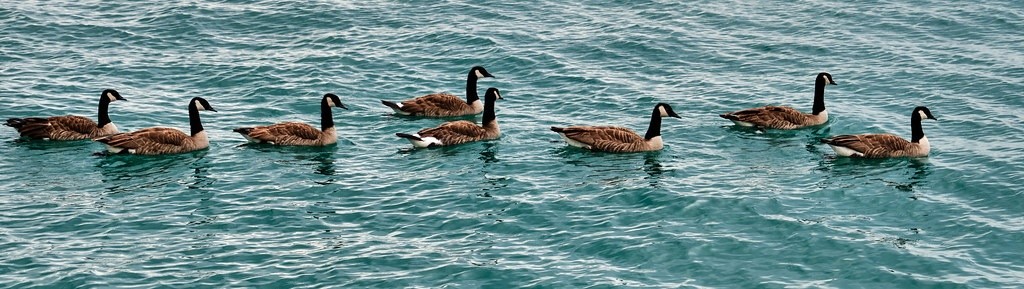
[2,90,129,141]
[718,73,838,129]
[396,87,504,148]
[91,97,217,156]
[381,66,495,117]
[232,94,348,146]
[817,106,937,158]
[550,103,683,154]
